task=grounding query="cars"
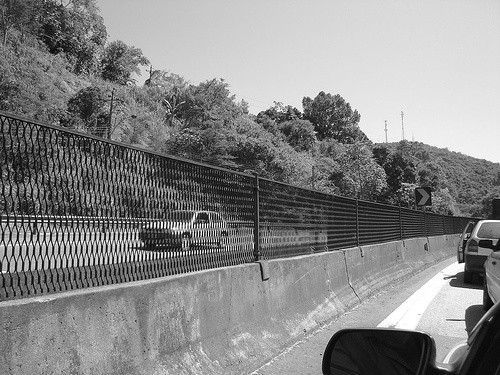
[457,219,500,284]
[138,209,230,251]
[478,238,500,310]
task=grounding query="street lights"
[104,89,137,157]
